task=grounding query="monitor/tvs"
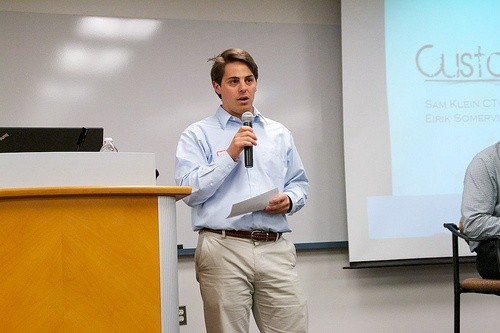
[0,127,104,153]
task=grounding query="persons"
[173,48,312,333]
[459,141,500,281]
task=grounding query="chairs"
[444,223,500,333]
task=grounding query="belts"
[203,226,284,242]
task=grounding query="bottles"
[99,137,119,152]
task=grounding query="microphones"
[241,112,254,168]
